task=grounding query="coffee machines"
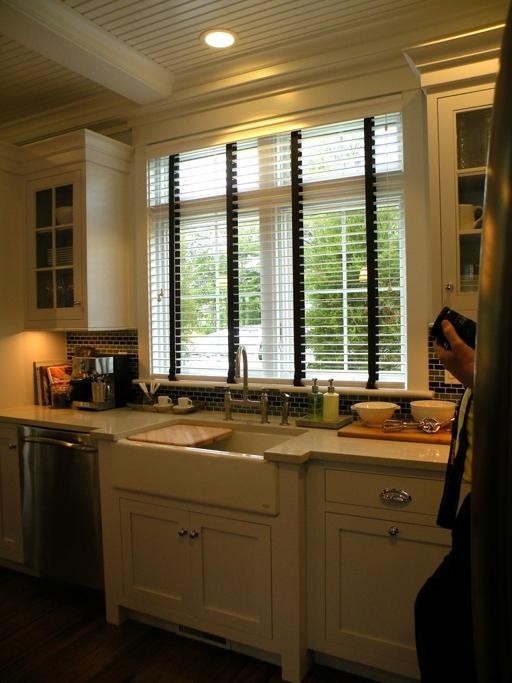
[92,354,132,411]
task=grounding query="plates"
[153,403,175,412]
[126,403,157,412]
[173,405,196,414]
[72,401,98,410]
[461,274,479,292]
[47,246,73,266]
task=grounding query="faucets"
[235,346,250,400]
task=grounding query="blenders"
[70,356,97,411]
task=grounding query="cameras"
[431,306,475,353]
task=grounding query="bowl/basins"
[55,206,74,224]
[409,399,457,428]
[350,401,401,427]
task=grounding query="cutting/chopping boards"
[337,419,452,444]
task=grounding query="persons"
[413,317,476,682]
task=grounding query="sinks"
[110,428,295,516]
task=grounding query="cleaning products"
[307,378,323,422]
[323,379,340,423]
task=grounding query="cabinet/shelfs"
[19,128,137,333]
[0,414,25,573]
[116,488,273,653]
[436,88,502,312]
[307,452,471,683]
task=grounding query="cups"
[178,396,192,407]
[458,204,484,230]
[158,396,172,405]
